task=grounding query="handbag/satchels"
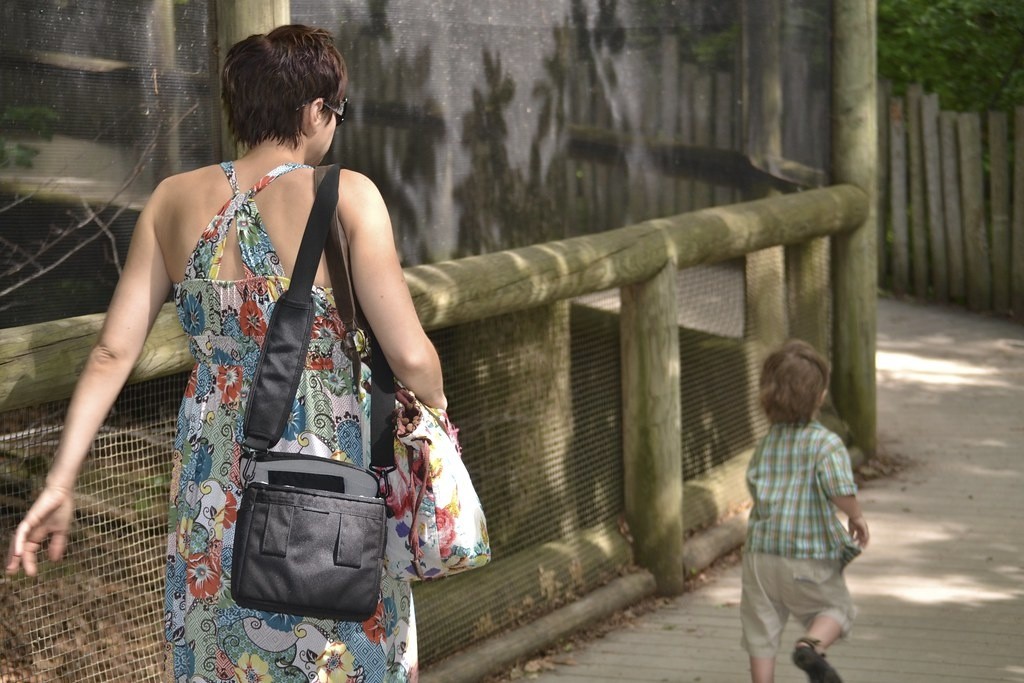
[231,451,386,618]
[339,328,492,582]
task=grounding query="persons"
[737,339,870,683]
[5,25,451,683]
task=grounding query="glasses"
[295,96,348,127]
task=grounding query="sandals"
[792,637,842,683]
